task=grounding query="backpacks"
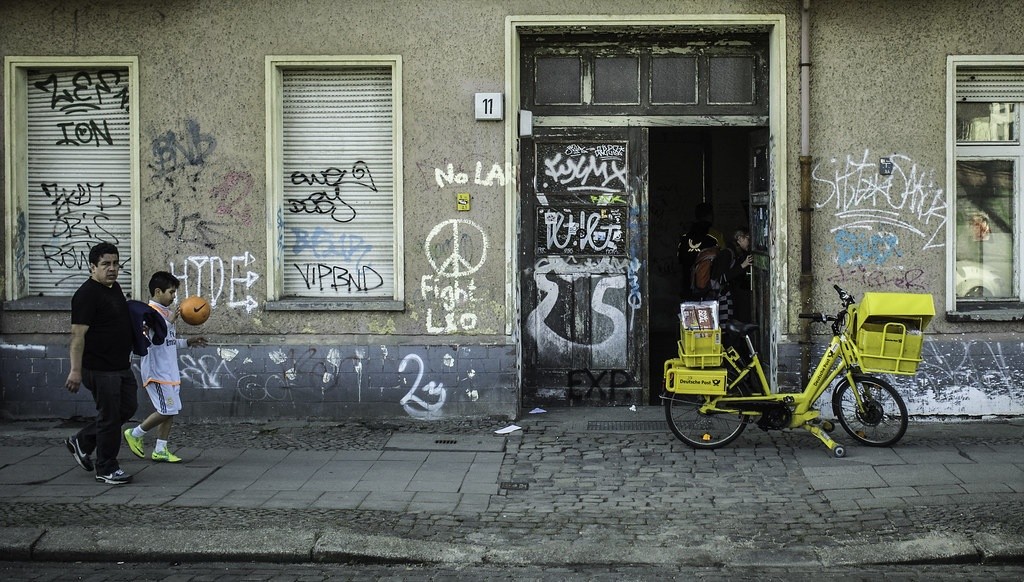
[689,244,736,300]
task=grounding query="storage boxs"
[856,291,936,373]
[677,312,721,367]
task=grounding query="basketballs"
[180,296,210,326]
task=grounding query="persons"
[700,226,754,359]
[63,242,149,485]
[123,270,209,463]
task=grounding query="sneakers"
[96,467,133,484]
[64,435,94,472]
[123,428,146,458]
[152,445,182,463]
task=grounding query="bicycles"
[658,285,936,458]
